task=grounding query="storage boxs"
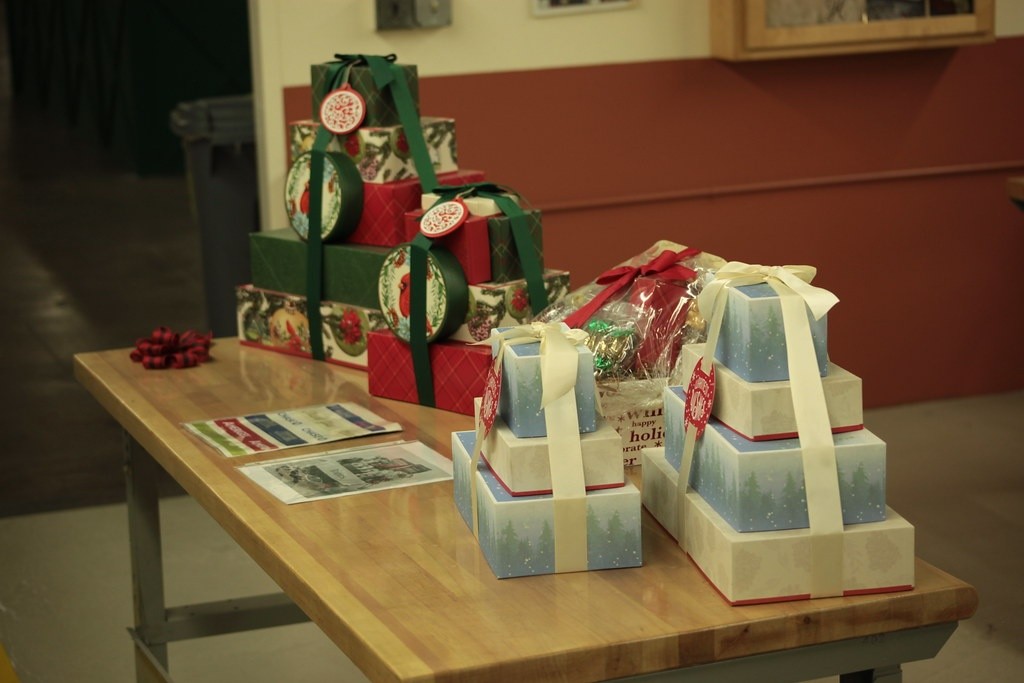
[474,396,625,497]
[401,201,524,288]
[641,447,917,606]
[447,267,570,345]
[662,383,889,533]
[310,61,421,129]
[708,282,828,382]
[599,378,680,489]
[487,323,603,439]
[234,283,386,372]
[680,342,865,442]
[367,328,496,417]
[246,227,394,310]
[341,169,484,248]
[288,120,459,184]
[452,429,643,581]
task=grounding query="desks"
[71,338,979,683]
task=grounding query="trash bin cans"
[170,94,262,338]
